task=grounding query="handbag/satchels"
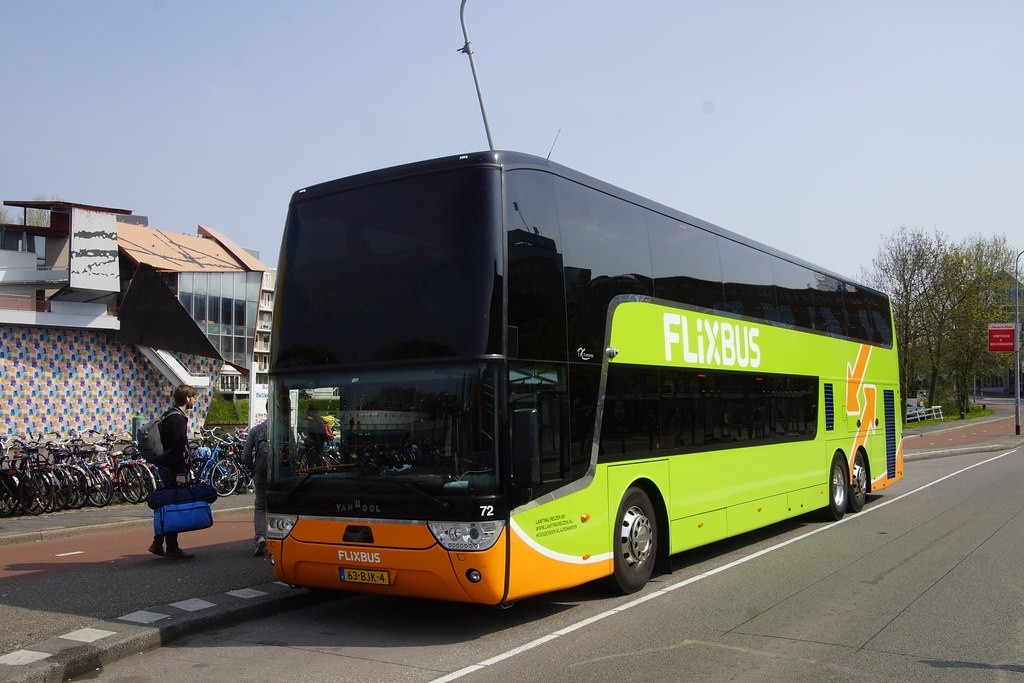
[322,418,333,438]
[153,502,213,535]
[147,484,218,509]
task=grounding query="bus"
[264,147,909,614]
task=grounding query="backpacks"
[254,424,268,478]
[137,411,182,464]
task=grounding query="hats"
[307,404,320,411]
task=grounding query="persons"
[300,401,330,471]
[148,384,198,559]
[242,402,269,557]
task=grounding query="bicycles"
[0,425,427,519]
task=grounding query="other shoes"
[254,537,266,555]
[148,543,165,556]
[165,544,195,560]
